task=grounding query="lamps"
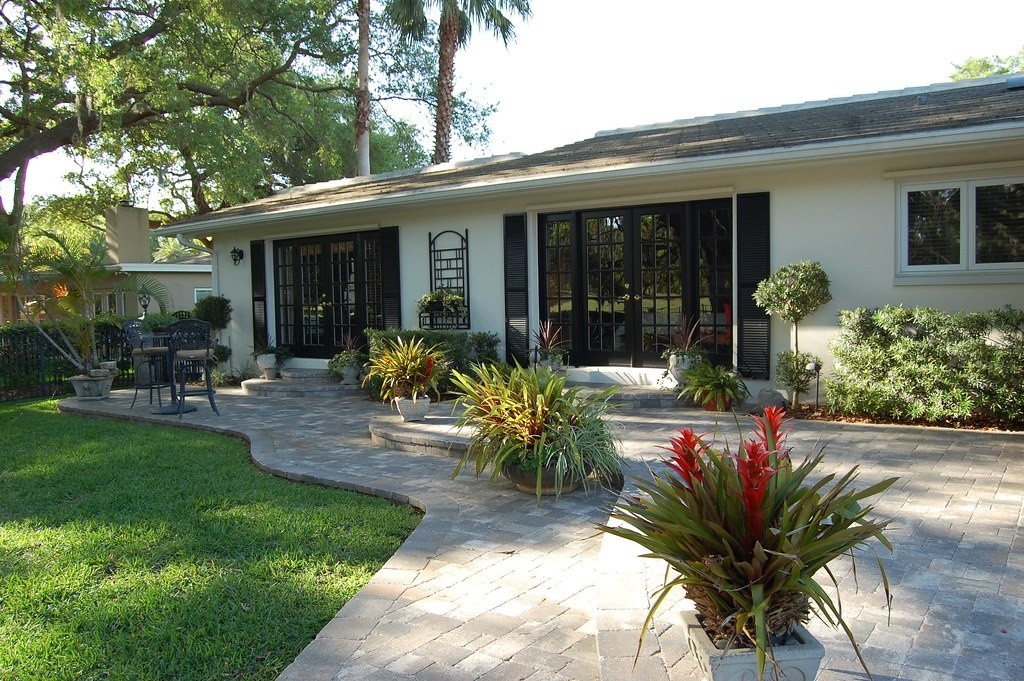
[137,284,151,309]
[230,246,244,266]
[805,362,822,373]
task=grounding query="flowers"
[586,404,901,681]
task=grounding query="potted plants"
[448,356,631,507]
[526,318,570,370]
[361,334,453,422]
[675,361,752,413]
[0,230,174,402]
[416,287,466,319]
[246,334,294,378]
[652,314,714,392]
[328,334,368,385]
[680,610,825,681]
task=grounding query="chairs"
[170,319,221,418]
[123,319,180,410]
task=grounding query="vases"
[264,368,278,380]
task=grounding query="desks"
[139,331,211,415]
